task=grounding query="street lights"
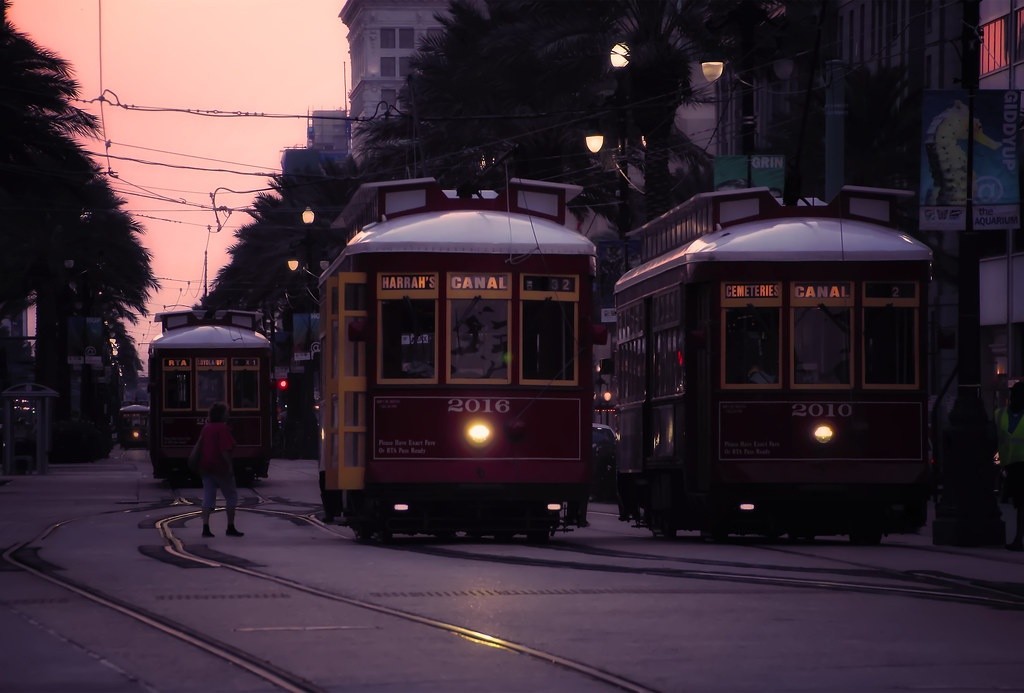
[610,41,636,239]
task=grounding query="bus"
[145,309,275,484]
[613,182,937,544]
[119,404,148,450]
[317,176,619,550]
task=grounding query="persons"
[990,382,1024,552]
[198,403,244,537]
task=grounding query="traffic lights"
[279,378,288,393]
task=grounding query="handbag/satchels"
[187,436,202,475]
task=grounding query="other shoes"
[225,529,245,537]
[201,532,216,538]
